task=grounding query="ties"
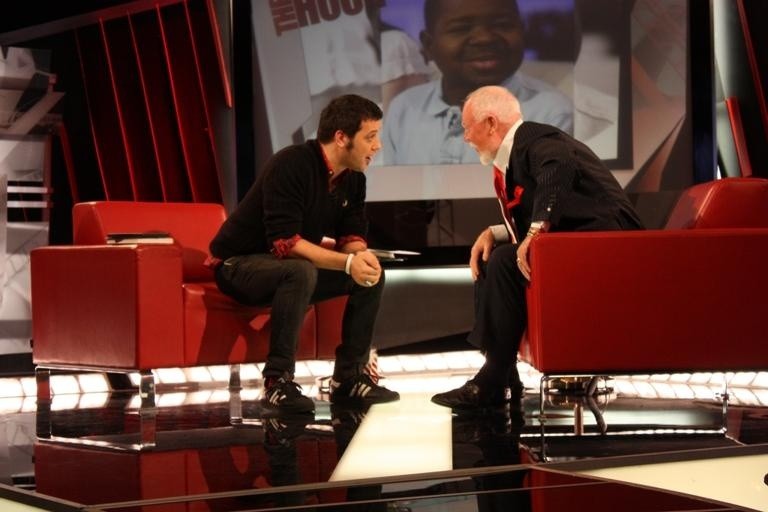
[492,166,526,244]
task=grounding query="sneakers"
[261,414,315,442]
[329,404,370,431]
[329,372,400,404]
[261,378,315,414]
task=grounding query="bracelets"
[525,227,540,239]
[345,250,354,276]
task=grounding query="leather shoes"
[431,380,526,409]
[452,411,526,442]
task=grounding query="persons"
[454,413,537,511]
[208,92,400,416]
[378,2,575,165]
[430,85,647,413]
[258,408,390,512]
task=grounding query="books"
[103,232,175,247]
[369,244,422,263]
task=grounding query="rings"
[515,257,522,263]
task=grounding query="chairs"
[518,175,768,466]
[27,203,350,452]
